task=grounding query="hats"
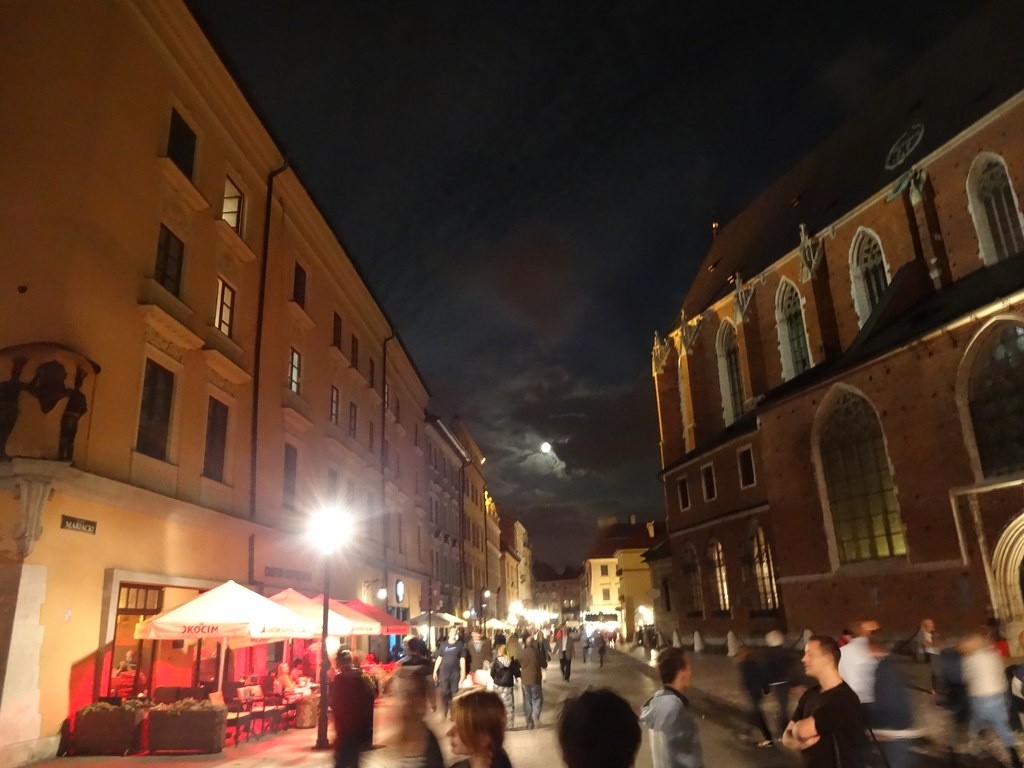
[765,630,784,647]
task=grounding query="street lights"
[305,498,357,751]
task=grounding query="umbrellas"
[134,579,314,702]
[267,587,519,667]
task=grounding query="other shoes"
[758,740,773,747]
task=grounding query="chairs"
[154,669,320,748]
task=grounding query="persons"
[355,667,445,768]
[328,648,368,767]
[116,650,136,677]
[288,617,659,752]
[555,684,643,768]
[639,645,707,768]
[731,609,1024,768]
[446,689,513,768]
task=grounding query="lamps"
[364,578,387,600]
